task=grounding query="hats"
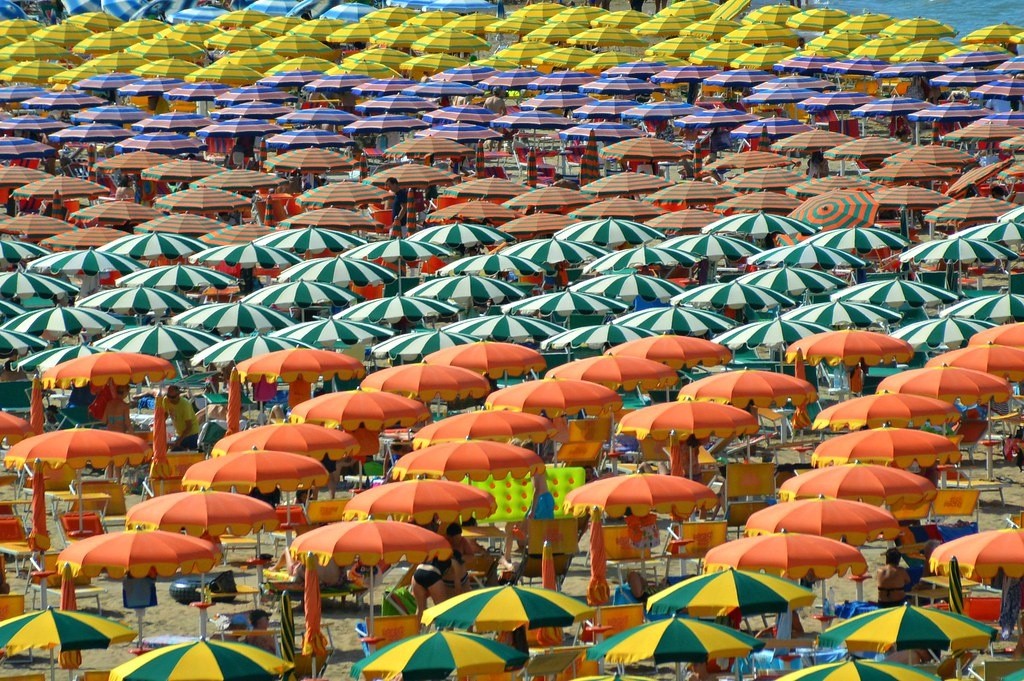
[249,610,272,622]
[231,615,254,629]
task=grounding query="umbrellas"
[0,1,1024,681]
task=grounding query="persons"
[162,385,201,452]
[101,383,133,486]
[875,547,909,608]
[411,547,462,630]
[439,521,474,599]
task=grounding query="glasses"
[166,394,178,399]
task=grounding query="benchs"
[0,94,1024,681]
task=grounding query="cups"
[1013,386,1019,396]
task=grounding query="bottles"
[337,475,359,493]
[833,365,840,388]
[120,456,154,494]
[359,475,363,489]
[828,586,835,616]
[823,598,830,616]
[129,417,154,432]
[285,408,291,424]
[366,475,370,489]
